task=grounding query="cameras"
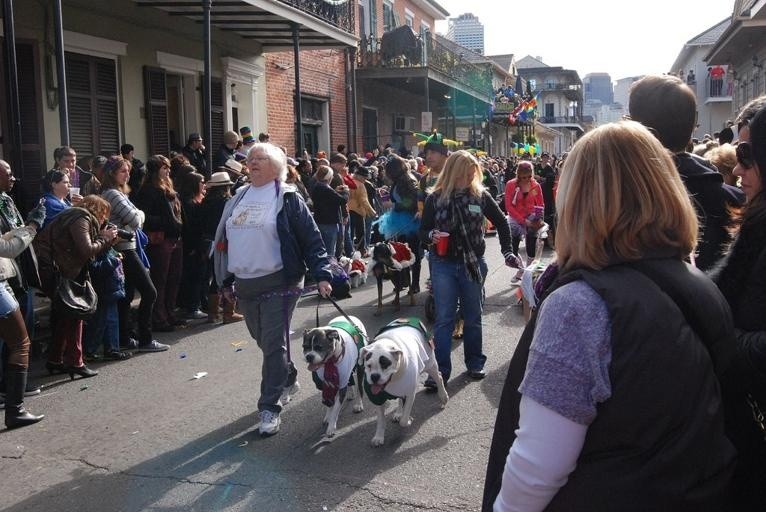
[101,220,136,242]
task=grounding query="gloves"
[504,250,520,267]
[26,206,45,230]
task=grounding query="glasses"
[736,143,755,169]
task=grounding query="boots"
[207,294,223,323]
[222,294,243,324]
[5,368,44,427]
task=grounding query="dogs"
[371,238,416,316]
[302,316,369,438]
[358,317,449,449]
[338,254,368,288]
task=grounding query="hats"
[412,129,462,157]
[188,126,255,185]
[356,167,368,176]
[103,156,122,174]
[287,157,300,166]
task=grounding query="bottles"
[216,241,228,254]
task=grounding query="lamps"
[751,54,761,67]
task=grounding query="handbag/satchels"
[52,279,97,318]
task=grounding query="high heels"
[67,363,97,380]
[45,363,68,375]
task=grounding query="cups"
[436,231,450,255]
[68,188,79,203]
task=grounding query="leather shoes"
[424,372,446,388]
[467,368,484,379]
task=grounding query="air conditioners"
[393,114,416,135]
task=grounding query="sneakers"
[103,308,207,361]
[258,410,280,437]
[279,382,301,406]
[23,385,40,396]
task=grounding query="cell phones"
[36,199,42,208]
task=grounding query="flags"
[507,89,539,124]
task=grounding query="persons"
[168,128,269,330]
[690,117,746,191]
[286,128,462,295]
[1,143,188,377]
[0,198,47,429]
[468,149,570,283]
[421,150,521,387]
[705,94,763,509]
[211,139,336,438]
[494,84,534,106]
[662,63,734,99]
[624,73,744,277]
[478,120,752,510]
